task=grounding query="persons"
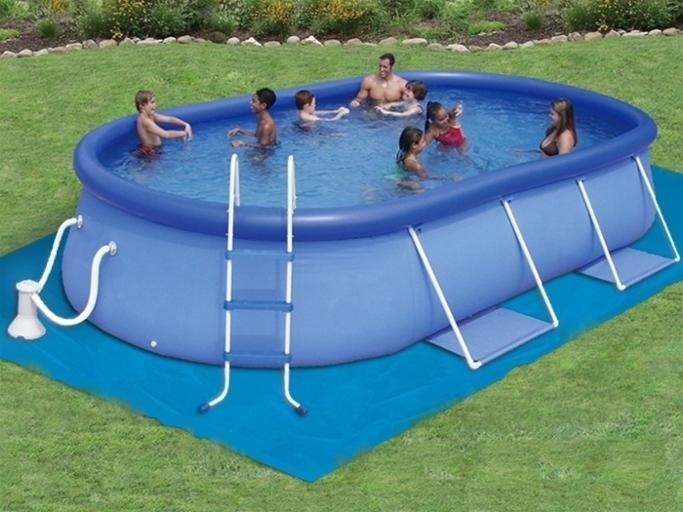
[391,126,451,191]
[424,99,464,151]
[133,89,193,158]
[352,53,411,109]
[293,90,351,132]
[225,88,277,150]
[537,96,577,160]
[374,79,427,120]
